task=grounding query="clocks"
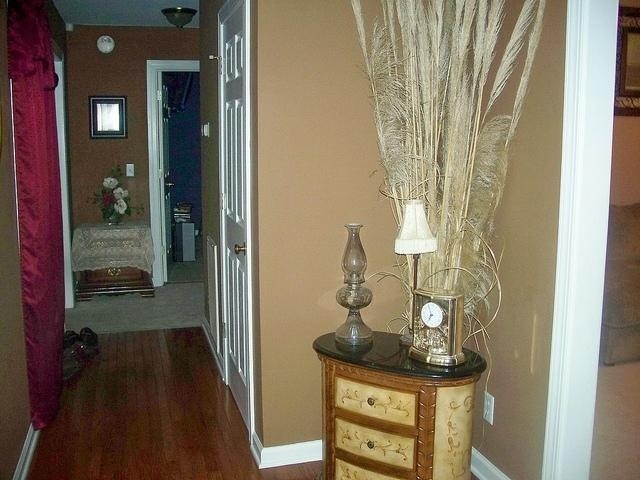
[407,266,481,367]
[95,33,116,55]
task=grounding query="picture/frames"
[89,95,128,140]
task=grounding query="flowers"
[92,160,132,218]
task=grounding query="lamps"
[393,202,438,348]
[161,7,197,30]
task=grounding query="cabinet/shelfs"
[71,223,154,302]
[314,328,487,480]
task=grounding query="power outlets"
[482,390,495,427]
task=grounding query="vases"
[105,218,120,226]
